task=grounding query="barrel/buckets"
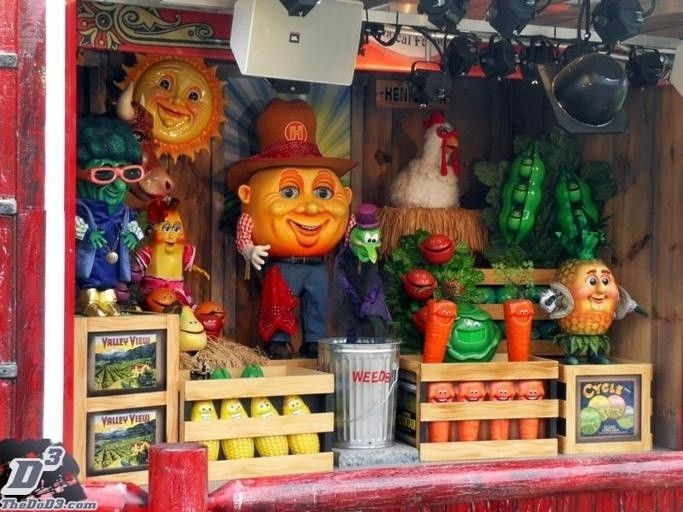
[315,336,403,450]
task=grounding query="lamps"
[223,0,683,138]
